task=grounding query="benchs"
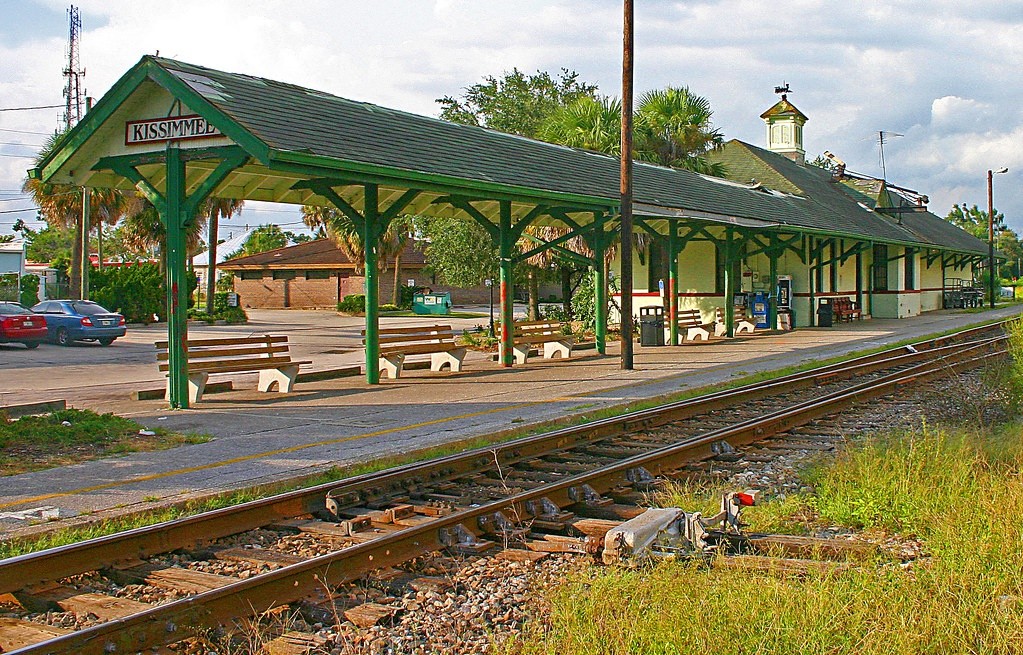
[360,326,473,380]
[714,306,761,337]
[662,309,710,346]
[494,320,572,366]
[831,297,863,323]
[155,334,312,403]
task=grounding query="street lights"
[987,166,1009,308]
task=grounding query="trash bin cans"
[411,287,452,309]
[640,305,665,347]
[817,298,834,327]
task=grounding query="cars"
[30,299,127,347]
[0,301,48,350]
